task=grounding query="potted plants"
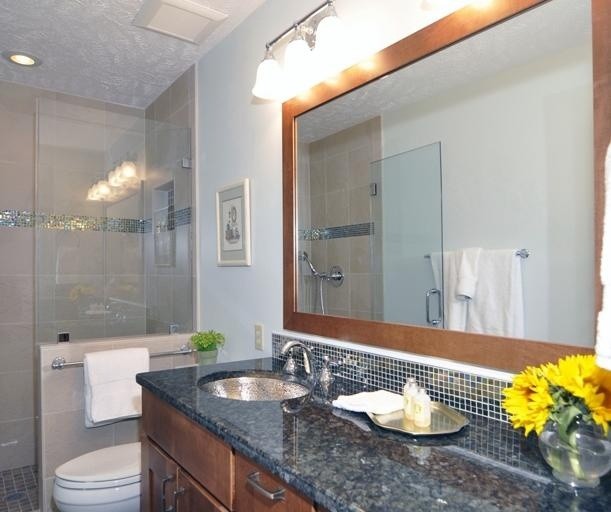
[191,329,225,366]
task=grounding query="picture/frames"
[215,178,251,266]
[151,177,176,266]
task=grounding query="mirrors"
[282,0,611,375]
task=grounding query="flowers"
[500,354,611,478]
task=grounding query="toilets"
[53,440,142,511]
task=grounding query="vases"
[538,419,611,487]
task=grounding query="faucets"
[278,340,316,381]
[280,382,314,416]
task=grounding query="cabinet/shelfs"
[139,387,235,512]
[234,452,316,512]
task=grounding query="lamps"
[252,0,341,99]
[85,156,136,200]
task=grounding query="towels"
[595,144,610,371]
[332,387,404,415]
[431,247,523,338]
[83,348,149,428]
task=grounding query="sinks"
[195,368,314,402]
